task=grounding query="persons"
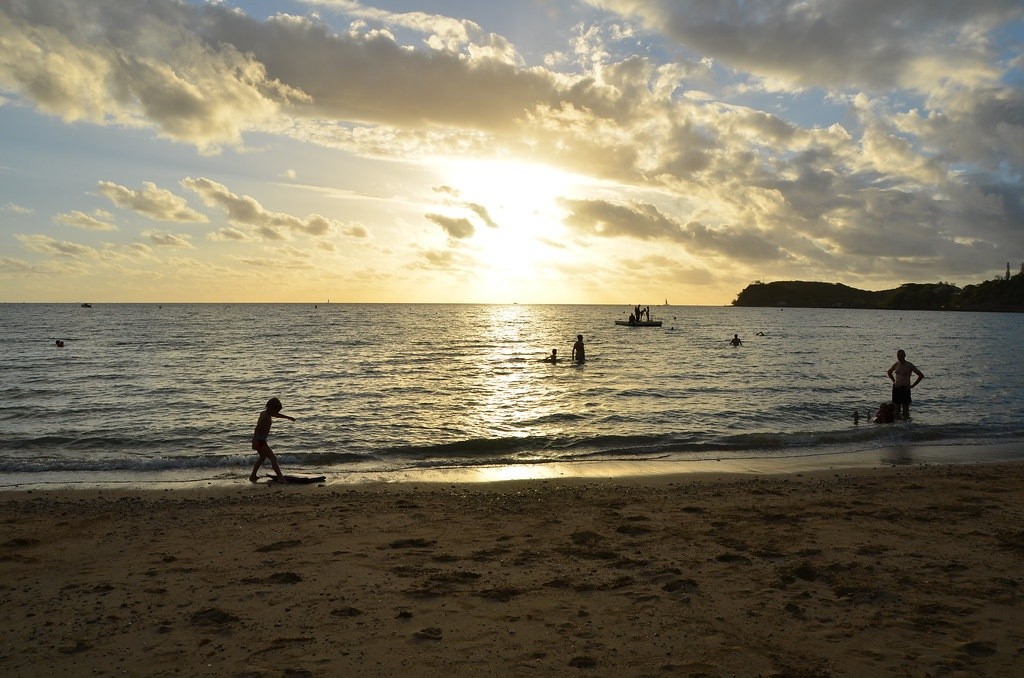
[730,334,742,345]
[545,349,557,364]
[572,334,585,364]
[629,305,650,323]
[249,398,297,486]
[887,349,925,419]
[756,331,765,335]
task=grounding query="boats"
[614,319,662,327]
